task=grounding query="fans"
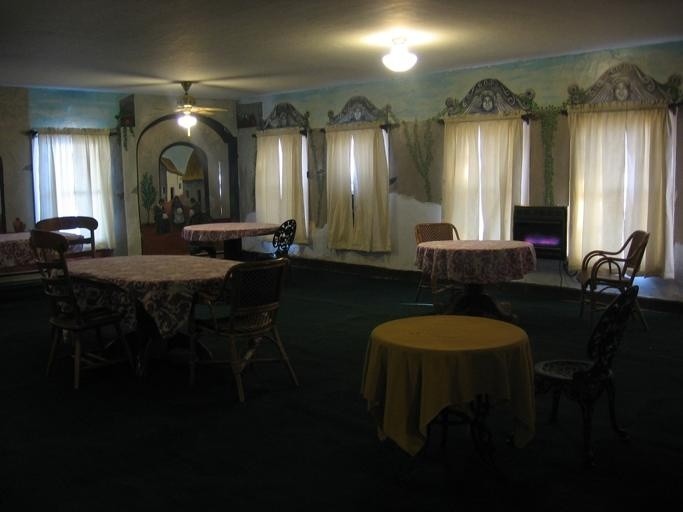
[146,83,228,116]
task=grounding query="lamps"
[177,84,197,128]
[381,33,418,72]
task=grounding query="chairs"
[34,217,98,259]
[29,229,69,277]
[273,219,296,287]
[188,258,300,403]
[29,232,133,390]
[532,284,640,439]
[576,230,650,335]
[413,222,460,301]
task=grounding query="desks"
[414,240,536,322]
[181,223,280,261]
[359,314,536,458]
[0,233,47,275]
[70,255,245,384]
